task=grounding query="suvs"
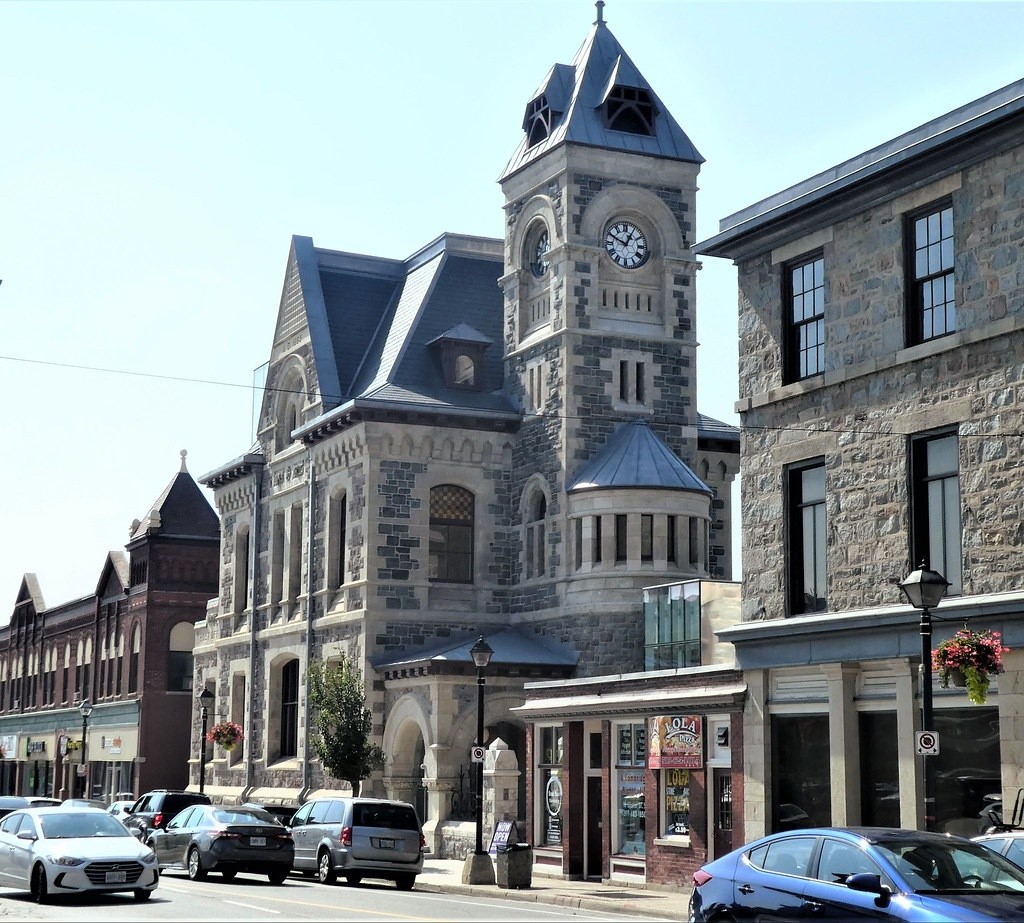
[122,789,211,843]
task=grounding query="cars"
[0,807,157,905]
[950,827,1024,891]
[62,799,109,812]
[144,803,295,886]
[688,825,1024,923]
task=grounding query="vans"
[288,797,425,891]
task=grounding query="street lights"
[79,698,93,799]
[470,635,494,855]
[898,559,955,831]
[195,686,216,793]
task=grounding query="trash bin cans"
[496,842,534,889]
